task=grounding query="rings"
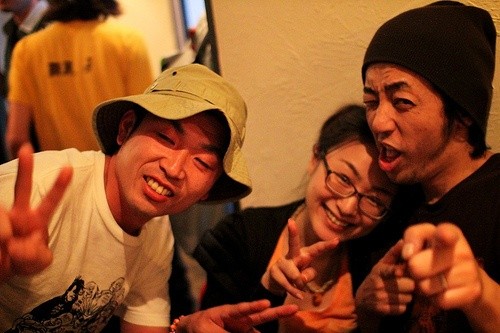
[439,273,449,291]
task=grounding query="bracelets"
[170,315,187,333]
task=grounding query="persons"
[190,105,401,333]
[0,62,298,333]
[5,0,155,161]
[0,0,55,78]
[354,0,500,333]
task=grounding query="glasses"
[321,155,392,222]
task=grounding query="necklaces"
[292,203,335,306]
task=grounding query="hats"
[92,63,254,206]
[362,0,498,134]
